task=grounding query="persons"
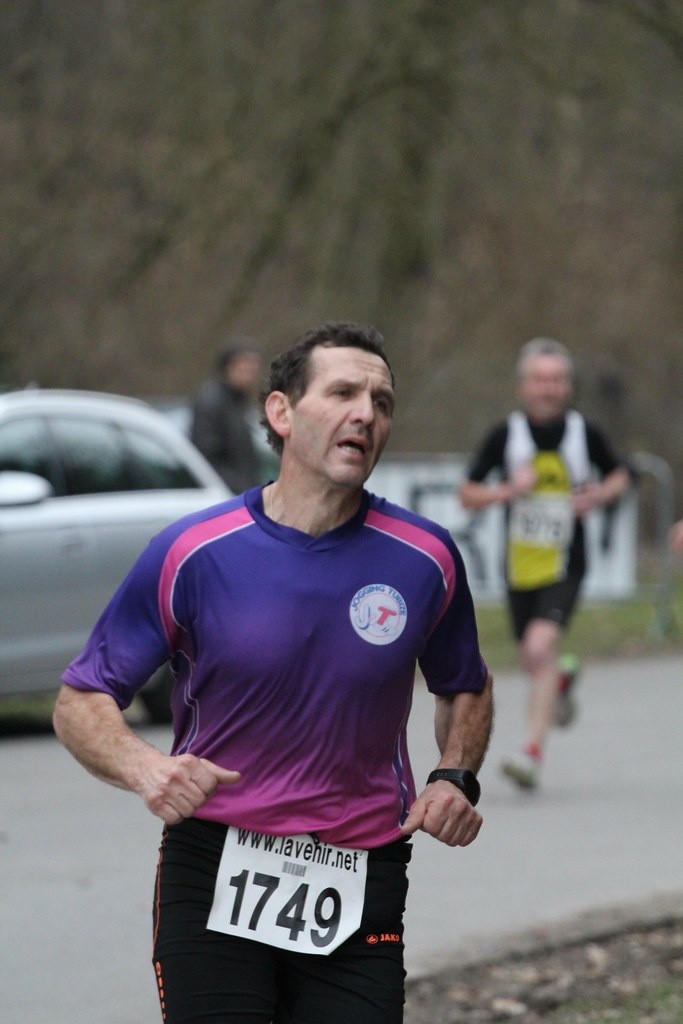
[190,338,260,495]
[53,323,493,1024]
[458,339,637,791]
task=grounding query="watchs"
[425,768,481,807]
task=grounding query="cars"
[0,379,244,727]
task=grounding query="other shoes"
[503,749,540,787]
[554,654,581,726]
